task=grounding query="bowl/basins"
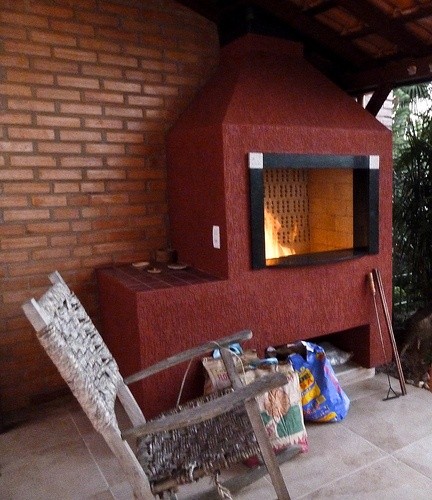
[132,262,150,271]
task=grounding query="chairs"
[22,269,302,500]
